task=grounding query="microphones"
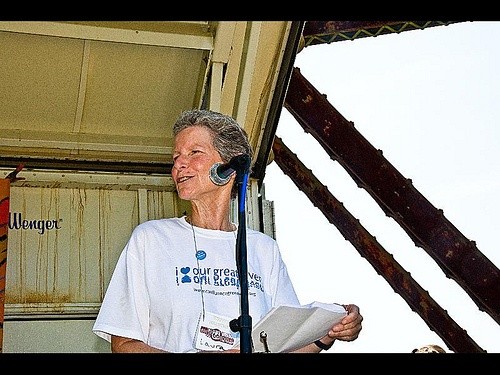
[209,156,243,185]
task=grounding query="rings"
[344,304,349,312]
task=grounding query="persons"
[92,106,363,354]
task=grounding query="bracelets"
[313,339,336,351]
